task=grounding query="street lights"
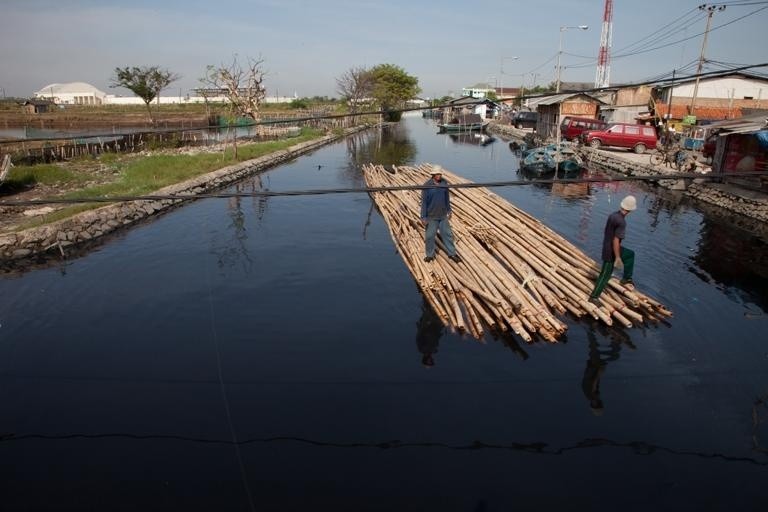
[556,25,588,94]
[500,56,519,123]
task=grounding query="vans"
[559,116,609,143]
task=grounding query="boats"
[422,115,442,119]
[359,162,676,345]
[422,111,442,116]
[436,130,490,136]
[437,122,489,131]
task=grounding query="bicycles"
[649,144,686,170]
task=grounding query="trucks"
[700,131,717,165]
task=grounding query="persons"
[669,124,676,131]
[416,296,443,369]
[420,166,461,263]
[582,317,624,416]
[588,195,636,307]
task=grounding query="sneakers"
[424,256,436,262]
[588,297,602,308]
[448,254,461,263]
[620,281,636,292]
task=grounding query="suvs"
[511,111,537,131]
[582,122,657,153]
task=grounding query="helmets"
[620,195,637,211]
[430,164,443,174]
